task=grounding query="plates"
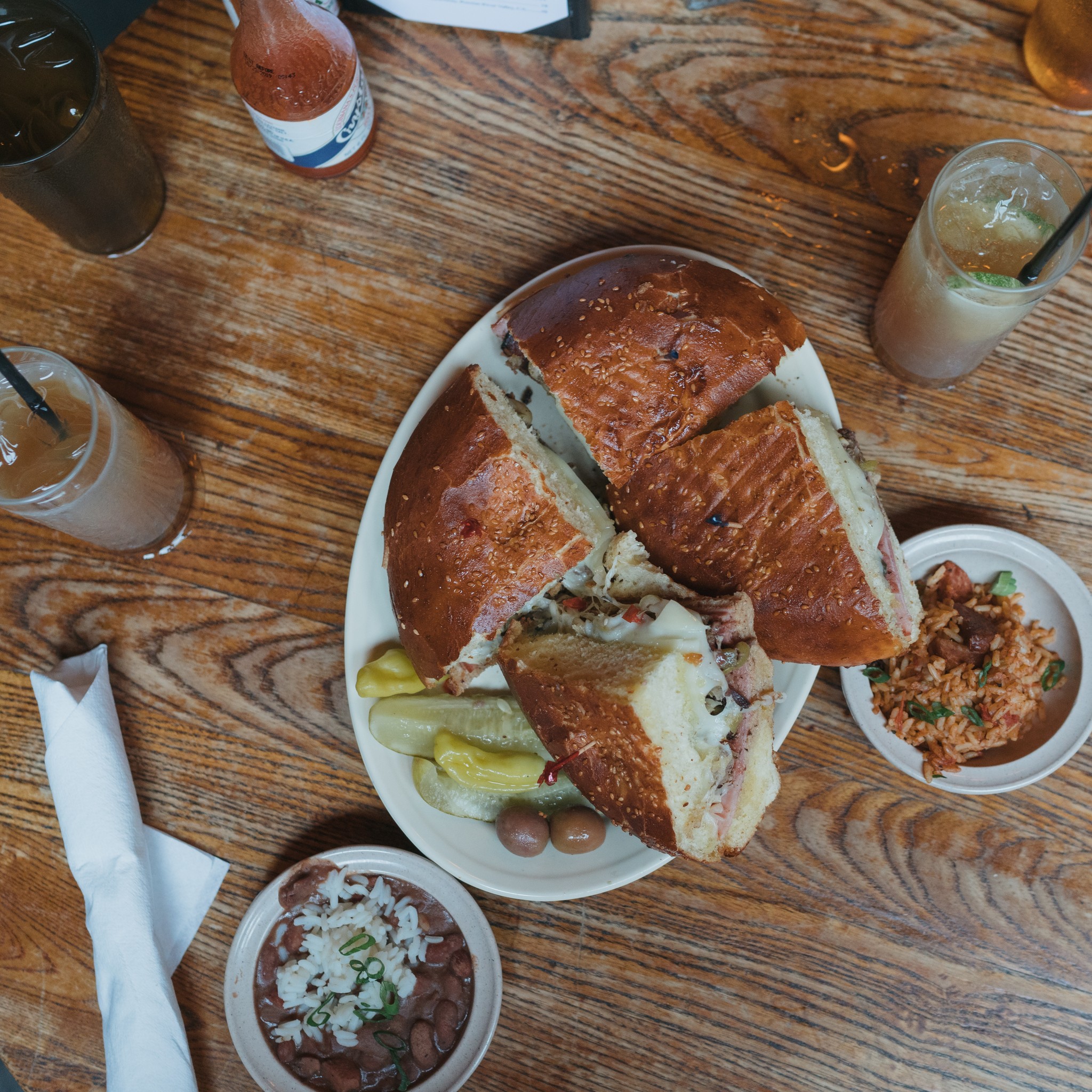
[226,843,503,1092]
[346,244,848,906]
[835,522,1091,795]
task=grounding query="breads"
[378,244,929,870]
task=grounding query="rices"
[864,557,1077,781]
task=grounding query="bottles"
[229,1,382,180]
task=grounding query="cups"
[867,139,1092,386]
[1023,0,1092,117]
[1,345,200,554]
[1,16,166,260]
